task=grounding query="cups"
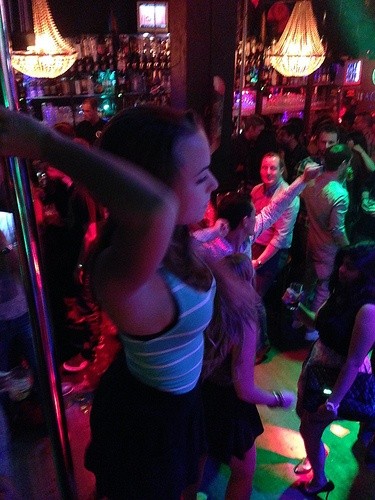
[38,102,73,124]
[235,90,304,111]
[286,283,303,310]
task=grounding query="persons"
[281,240,375,500]
[250,152,300,366]
[199,252,296,500]
[1,95,122,441]
[0,103,261,500]
[183,109,375,289]
[291,143,351,342]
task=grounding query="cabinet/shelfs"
[19,80,340,139]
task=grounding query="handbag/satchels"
[303,365,375,423]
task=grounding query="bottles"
[237,37,279,87]
[321,53,331,83]
[67,34,171,96]
[15,72,69,97]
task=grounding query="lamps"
[269,0,327,78]
[6,0,78,80]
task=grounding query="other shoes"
[255,344,271,364]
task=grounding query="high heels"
[297,478,334,500]
[294,442,329,474]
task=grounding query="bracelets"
[267,389,285,409]
[324,398,339,412]
